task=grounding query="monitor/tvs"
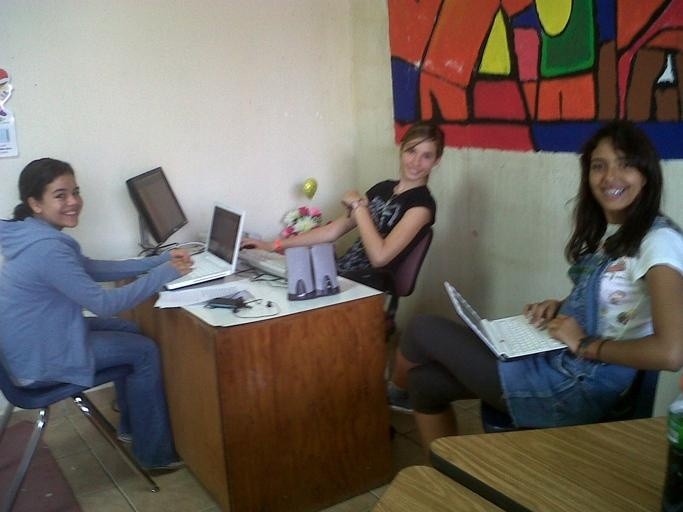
[125,167,189,246]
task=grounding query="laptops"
[444,281,569,361]
[159,203,245,289]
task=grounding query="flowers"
[279,208,325,239]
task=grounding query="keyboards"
[238,248,288,278]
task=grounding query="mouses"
[240,244,256,250]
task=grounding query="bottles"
[666,379,683,454]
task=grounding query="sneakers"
[386,382,414,414]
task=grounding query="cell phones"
[209,298,241,308]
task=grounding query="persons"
[0,155,195,474]
[238,120,446,303]
[383,117,683,472]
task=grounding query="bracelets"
[348,196,365,214]
[272,237,286,253]
[575,334,608,364]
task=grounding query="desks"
[114,255,391,511]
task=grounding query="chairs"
[0,362,159,511]
[336,225,433,340]
[481,366,660,434]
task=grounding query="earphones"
[268,301,272,307]
[234,308,238,314]
